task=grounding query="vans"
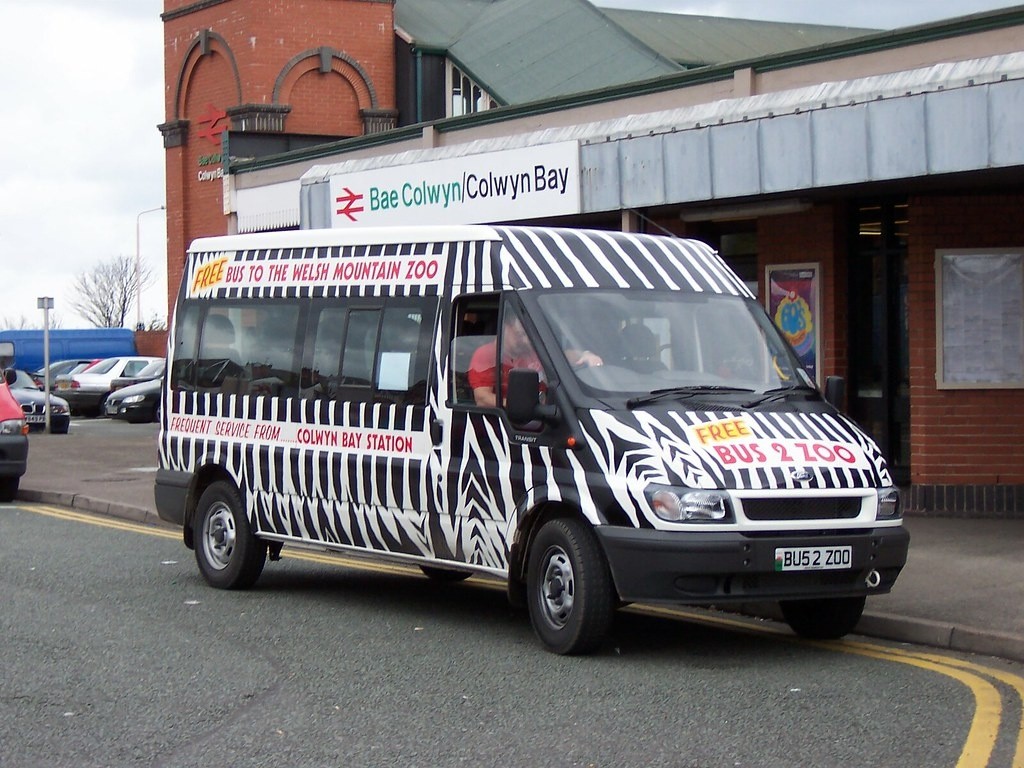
[151,221,910,656]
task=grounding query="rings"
[596,363,600,366]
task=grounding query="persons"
[468,305,604,428]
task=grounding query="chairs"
[197,316,502,405]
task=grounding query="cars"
[33,355,166,425]
[3,368,71,434]
[0,368,29,502]
